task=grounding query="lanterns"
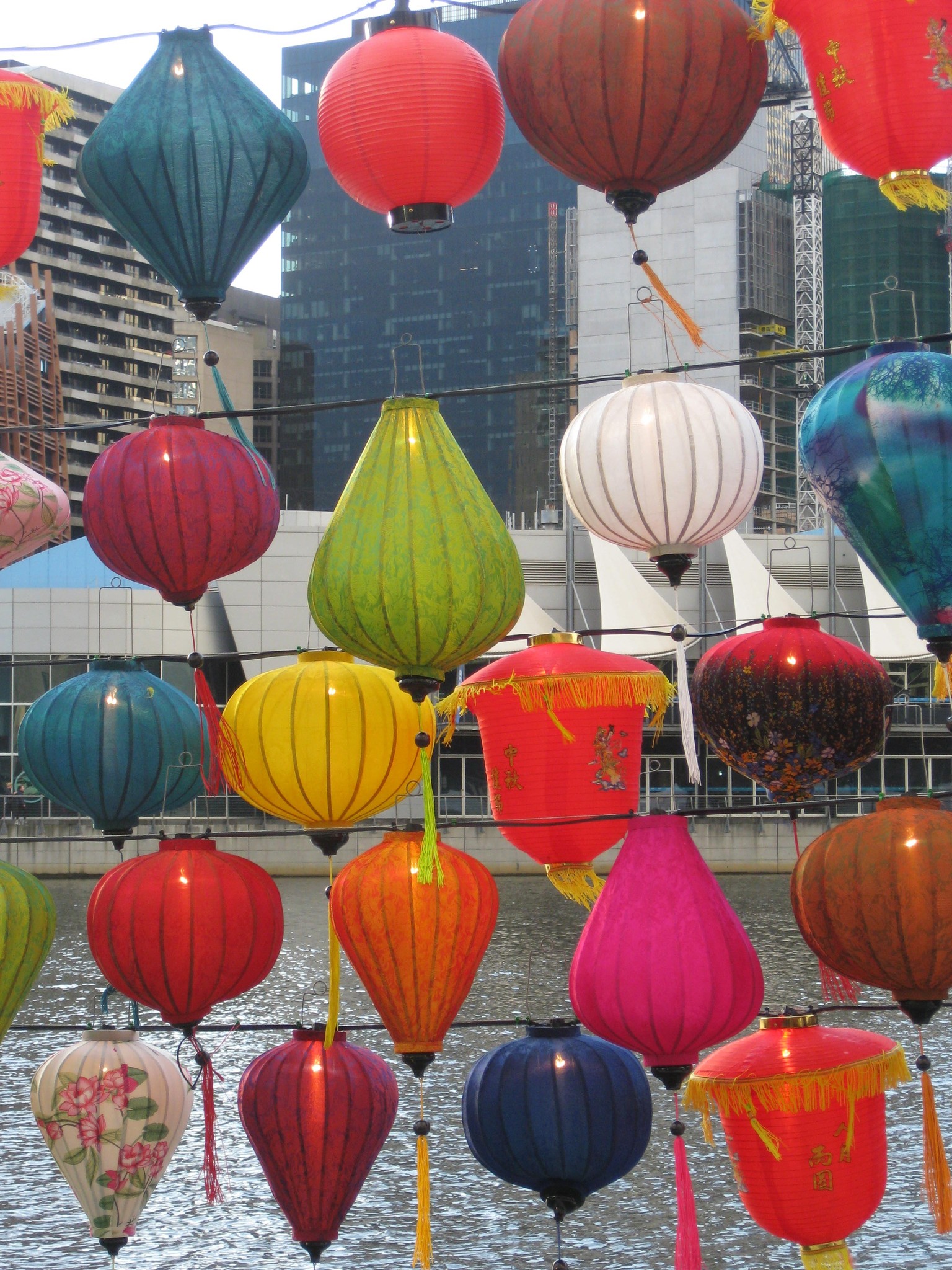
[77,24,310,487]
[747,0,952,214]
[317,7,505,235]
[0,69,78,268]
[0,350,952,1270]
[498,0,767,348]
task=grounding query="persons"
[3,782,16,819]
[16,785,26,819]
